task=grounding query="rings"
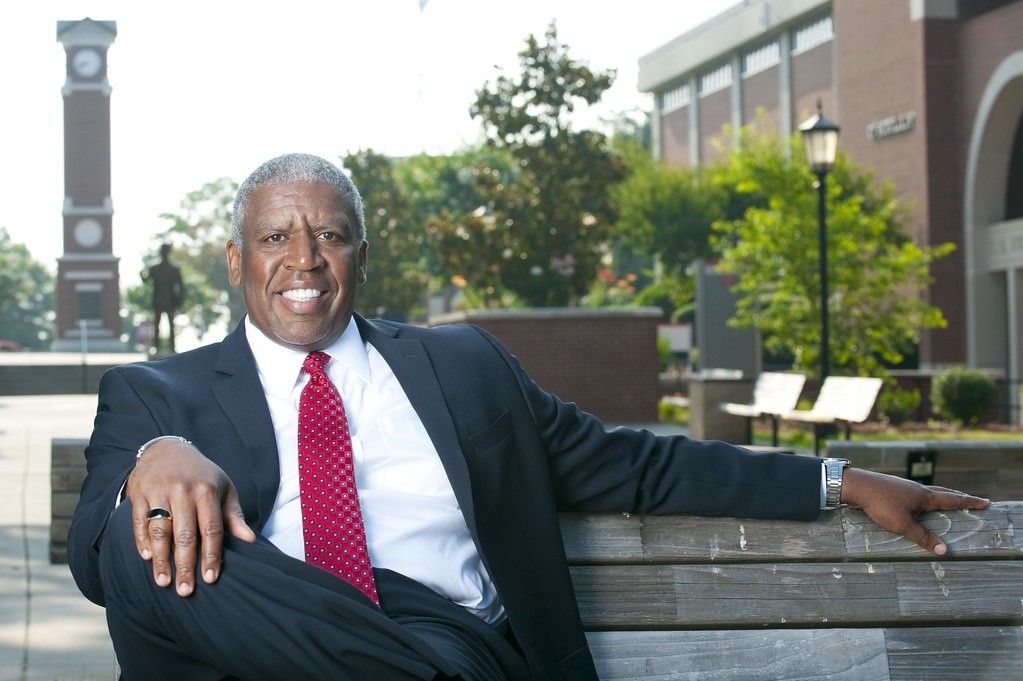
[148,507,173,521]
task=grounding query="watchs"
[823,458,851,508]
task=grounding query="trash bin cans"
[689,369,751,449]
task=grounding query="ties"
[298,352,382,610]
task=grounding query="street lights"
[797,97,840,384]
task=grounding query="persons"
[66,154,989,681]
[140,244,184,352]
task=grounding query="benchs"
[48,436,1023,681]
[719,371,806,448]
[781,376,884,455]
[827,437,1023,503]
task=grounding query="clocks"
[72,49,102,78]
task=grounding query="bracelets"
[136,436,193,466]
